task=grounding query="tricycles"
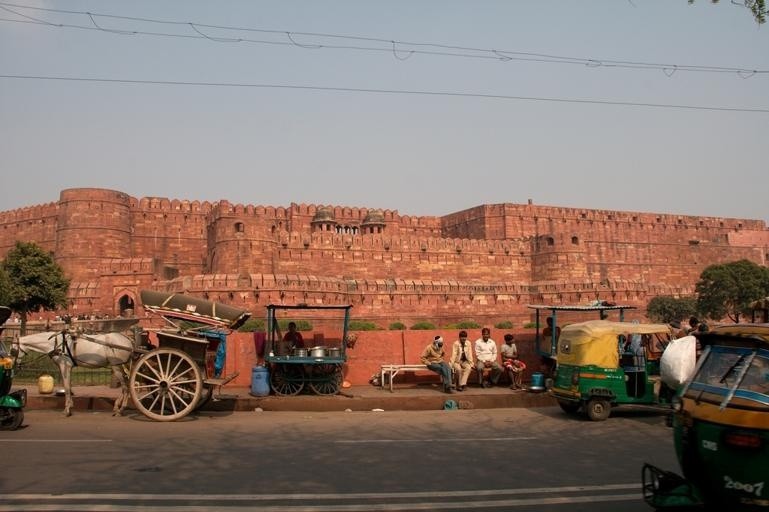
[641,324,768,509]
[0,335,26,430]
[546,322,677,421]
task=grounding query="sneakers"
[445,383,468,394]
[511,382,522,390]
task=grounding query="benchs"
[381,364,492,393]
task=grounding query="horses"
[7,330,134,417]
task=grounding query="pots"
[327,346,341,360]
[292,347,309,360]
[308,345,328,362]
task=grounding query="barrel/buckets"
[531,371,543,386]
[250,367,271,396]
[38,373,54,394]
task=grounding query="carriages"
[8,287,253,420]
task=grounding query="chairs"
[155,328,221,379]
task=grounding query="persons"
[474,328,505,387]
[682,315,707,336]
[499,334,528,390]
[419,335,458,394]
[542,316,561,352]
[448,331,477,391]
[698,323,708,331]
[282,320,304,354]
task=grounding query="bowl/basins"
[527,385,546,393]
[55,388,67,396]
